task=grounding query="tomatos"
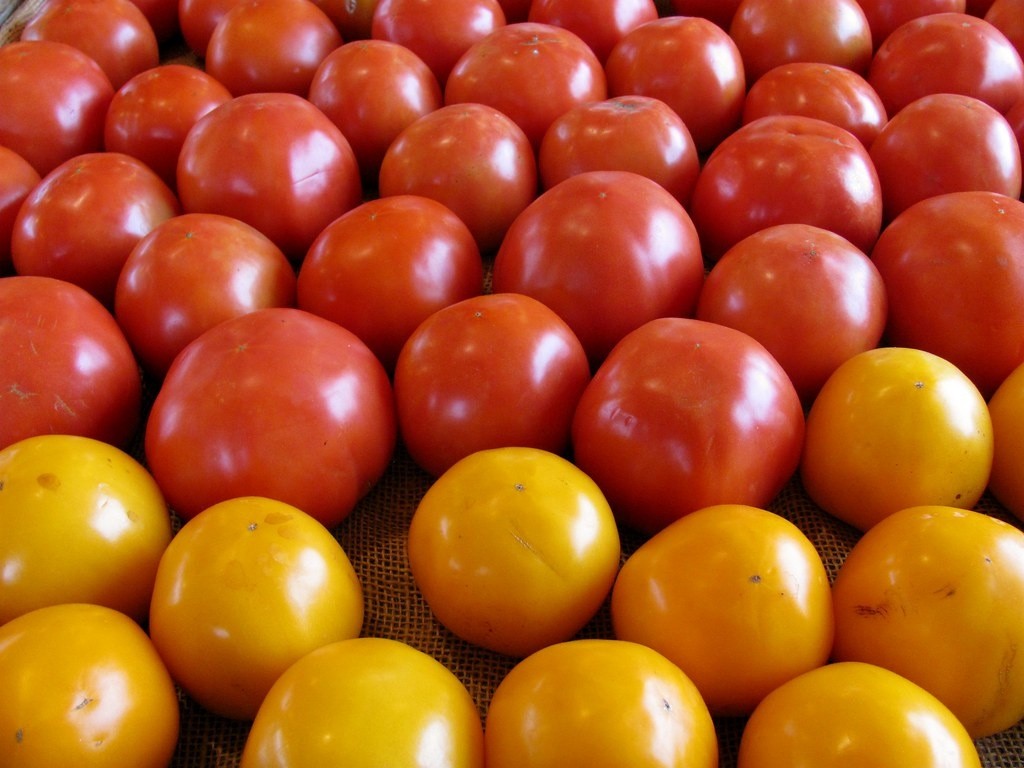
[0,1,1024,768]
